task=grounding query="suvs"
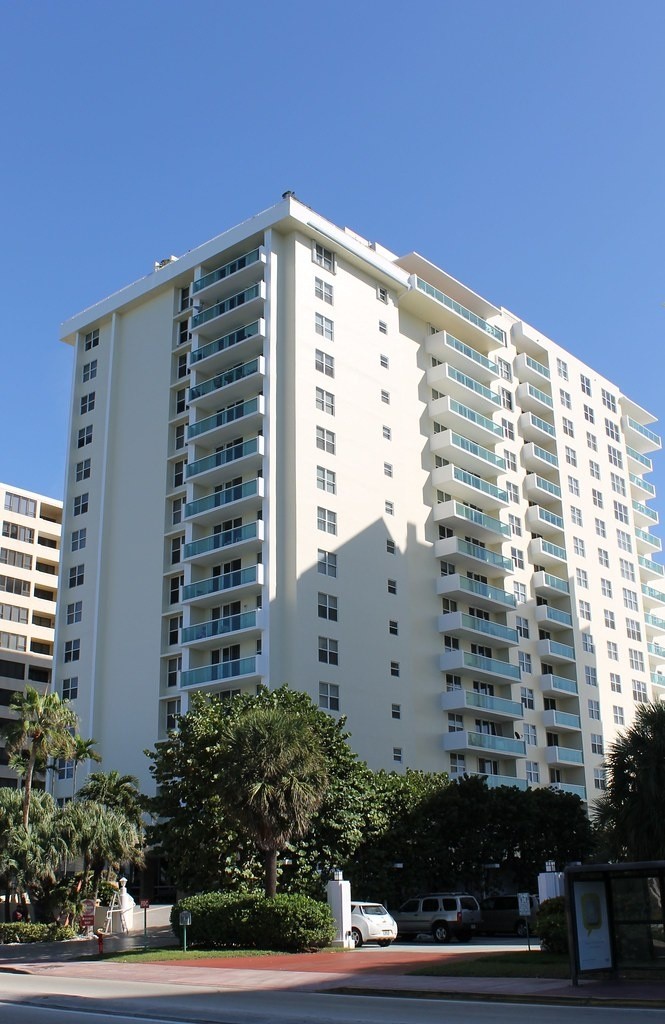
[350,901,398,948]
[386,892,481,942]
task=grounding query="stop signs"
[80,901,94,915]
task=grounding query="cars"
[478,893,541,937]
